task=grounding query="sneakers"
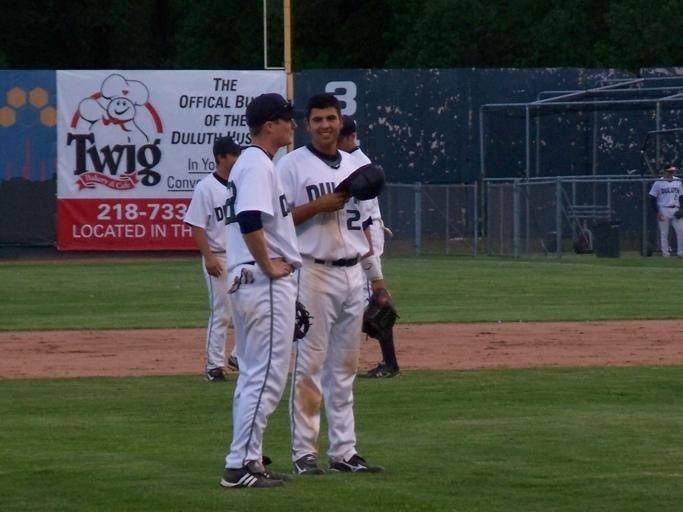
[366,358,402,384]
[249,463,293,481]
[205,366,223,382]
[219,468,284,489]
[226,355,243,373]
[293,453,328,479]
[328,451,387,474]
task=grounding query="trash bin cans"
[594,222,620,258]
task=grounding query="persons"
[649,164,683,258]
[218,93,302,489]
[277,92,391,474]
[182,138,249,385]
[337,115,401,378]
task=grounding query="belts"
[301,250,363,269]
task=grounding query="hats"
[210,136,249,154]
[663,164,676,172]
[339,112,355,143]
[332,162,385,206]
[245,91,308,123]
